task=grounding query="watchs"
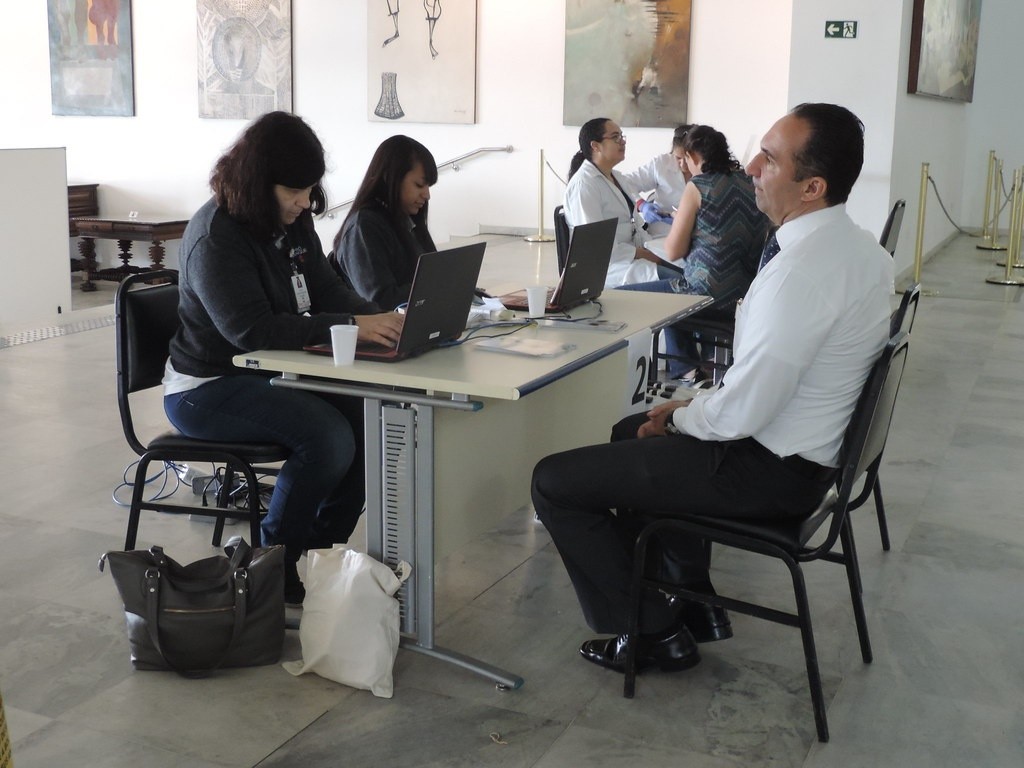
[664,407,681,436]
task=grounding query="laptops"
[302,241,486,362]
[491,216,618,312]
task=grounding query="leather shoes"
[579,625,701,674]
[667,595,733,642]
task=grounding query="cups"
[330,324,359,367]
[526,286,549,319]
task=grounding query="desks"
[232,281,715,692]
[70,214,193,293]
[67,183,100,272]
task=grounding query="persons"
[161,114,405,628]
[562,118,716,386]
[333,135,487,312]
[613,125,769,390]
[296,277,302,288]
[623,123,697,213]
[531,102,896,675]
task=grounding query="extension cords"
[191,473,241,495]
[189,507,239,525]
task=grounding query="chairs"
[553,205,570,278]
[115,269,290,556]
[623,199,921,744]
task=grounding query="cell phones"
[657,212,671,219]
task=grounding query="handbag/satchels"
[283,543,402,699]
[98,537,285,678]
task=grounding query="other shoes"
[690,369,712,390]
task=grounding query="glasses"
[596,136,627,144]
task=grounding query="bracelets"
[348,316,355,325]
